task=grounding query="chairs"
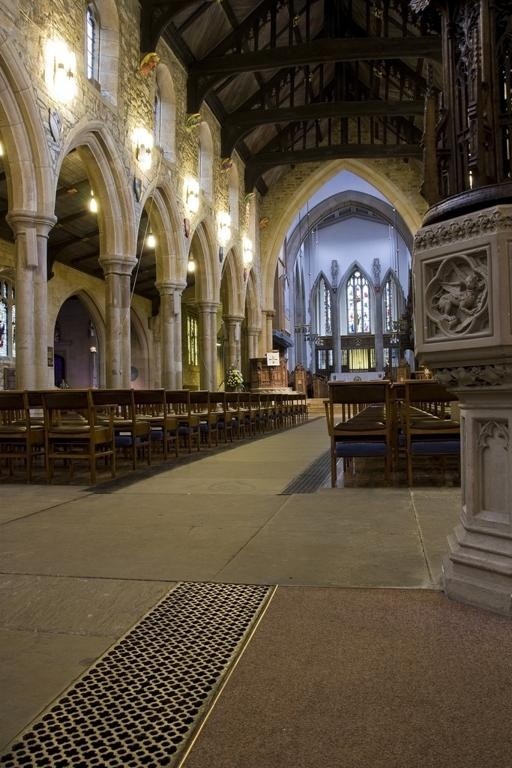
[322,377,460,488]
[0,389,309,484]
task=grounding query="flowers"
[216,355,248,391]
